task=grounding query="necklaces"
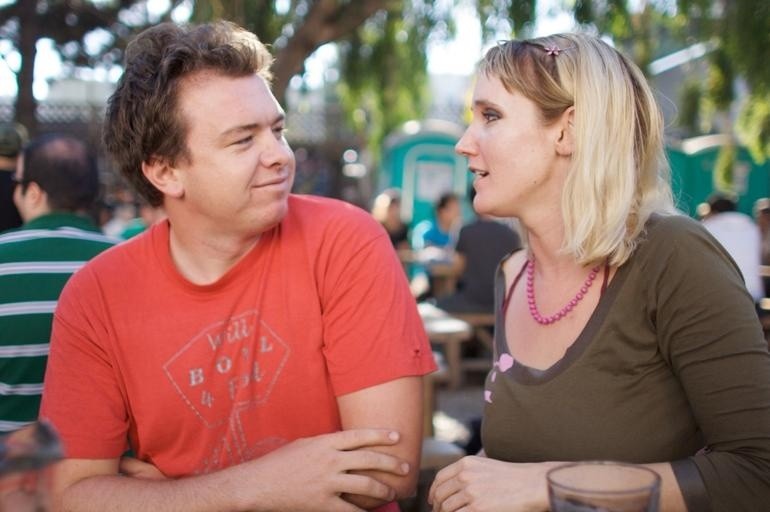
[526,253,604,324]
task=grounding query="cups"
[545,459,663,512]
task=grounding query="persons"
[0,123,27,238]
[37,20,438,511]
[1,134,123,443]
[750,197,770,301]
[369,184,522,312]
[426,37,770,512]
[686,190,764,309]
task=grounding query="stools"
[442,304,495,389]
